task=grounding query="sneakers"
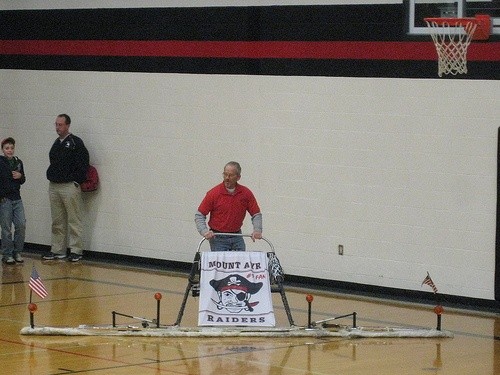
[42,252,66,258]
[68,254,83,261]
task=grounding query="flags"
[29,266,48,298]
[421,274,438,294]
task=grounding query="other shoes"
[5,257,15,265]
[15,253,24,262]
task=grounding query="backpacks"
[80,165,98,192]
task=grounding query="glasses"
[222,172,236,178]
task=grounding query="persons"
[195,161,262,252]
[41,114,89,262]
[0,138,26,265]
